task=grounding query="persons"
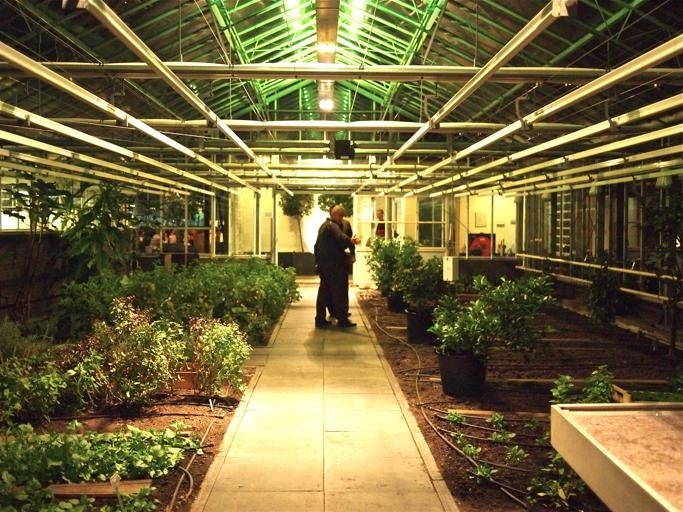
[374,207,385,241]
[325,206,354,318]
[311,205,362,328]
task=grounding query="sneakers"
[338,321,357,326]
[330,312,351,318]
[315,318,330,326]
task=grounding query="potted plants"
[365,233,500,399]
